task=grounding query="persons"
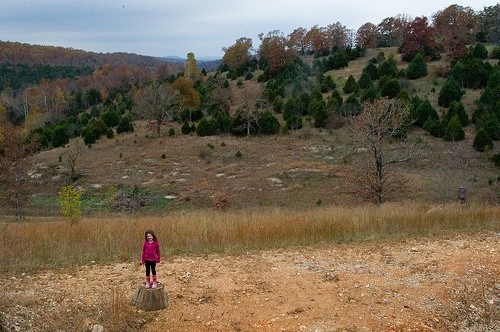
[140,229,160,289]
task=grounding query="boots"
[145,276,151,289]
[152,275,157,288]
